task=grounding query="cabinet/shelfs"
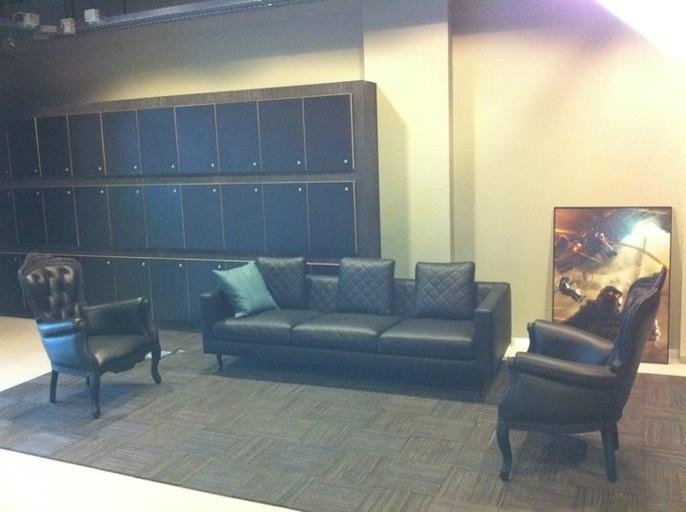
[0,81,381,329]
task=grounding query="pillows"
[414,262,475,319]
[257,256,306,309]
[334,257,395,314]
[213,260,277,319]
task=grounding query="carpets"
[0,331,686,512]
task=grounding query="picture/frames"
[552,205,672,365]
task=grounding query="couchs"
[200,274,511,390]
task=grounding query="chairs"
[17,253,161,418]
[496,267,666,481]
